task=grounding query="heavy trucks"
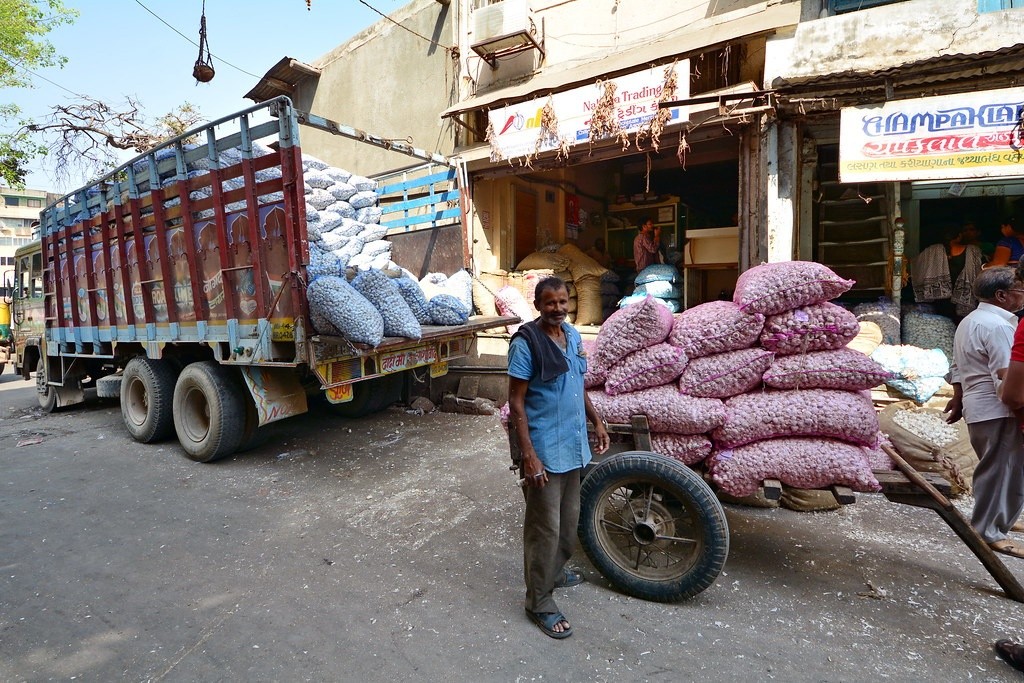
[3,95,522,463]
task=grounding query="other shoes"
[995,638,1024,674]
[985,539,1024,559]
[1009,521,1024,531]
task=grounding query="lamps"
[470,29,544,70]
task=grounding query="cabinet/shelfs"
[605,202,687,267]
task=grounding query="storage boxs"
[690,235,738,264]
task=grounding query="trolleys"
[505,413,1024,604]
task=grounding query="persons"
[912,215,1024,674]
[586,216,668,274]
[731,212,738,227]
[508,278,610,640]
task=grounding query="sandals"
[524,606,573,639]
[556,570,585,587]
